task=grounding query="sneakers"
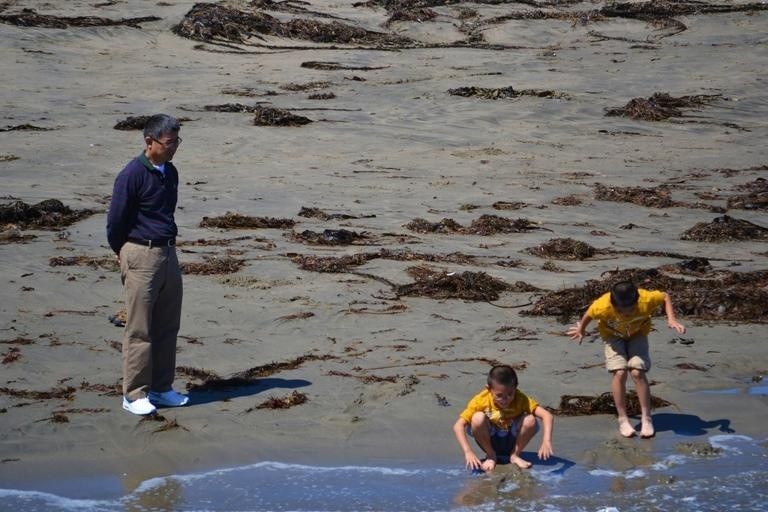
[123,395,156,415]
[148,390,190,407]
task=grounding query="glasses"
[150,136,182,149]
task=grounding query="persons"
[107,113,189,415]
[565,281,686,438]
[453,365,553,472]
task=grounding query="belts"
[127,237,176,247]
